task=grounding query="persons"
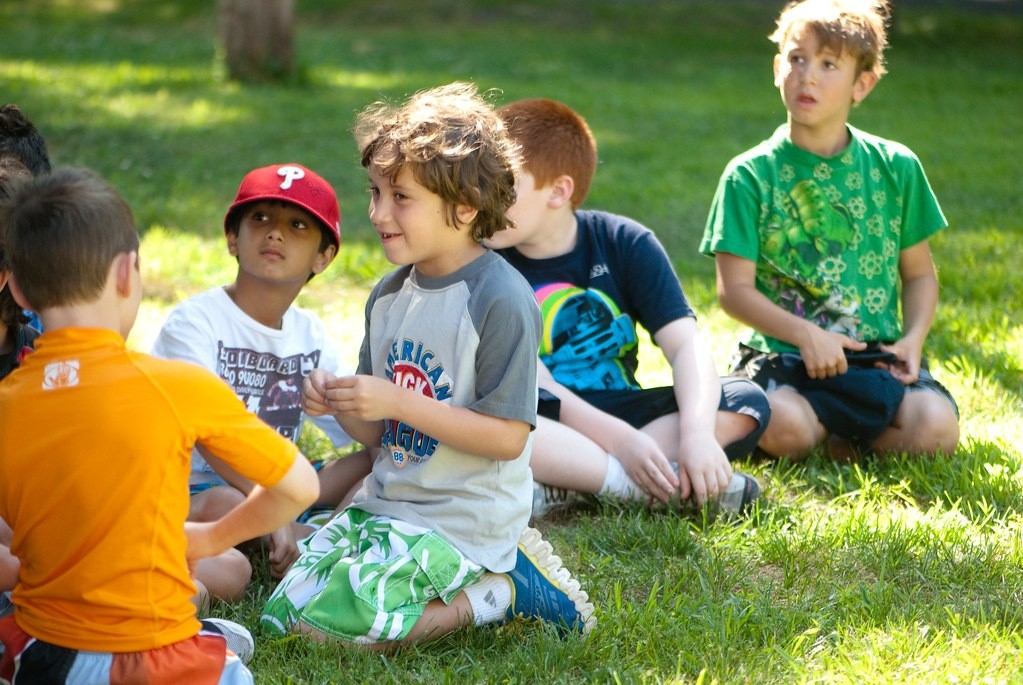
[0,103,321,685]
[698,0,961,462]
[477,100,770,518]
[150,164,379,578]
[262,82,597,651]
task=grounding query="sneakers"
[649,461,759,517]
[531,479,596,524]
[490,528,597,648]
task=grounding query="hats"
[225,163,341,282]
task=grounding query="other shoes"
[197,617,254,665]
